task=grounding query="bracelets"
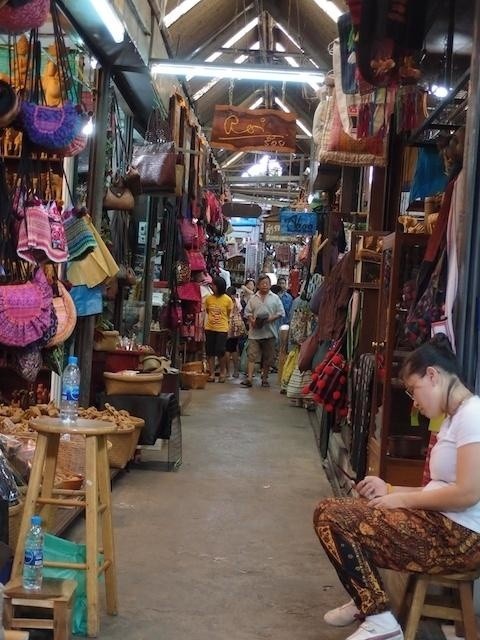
[386,483,393,493]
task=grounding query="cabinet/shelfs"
[366,232,447,486]
[149,281,172,355]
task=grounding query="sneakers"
[207,375,271,388]
[346,612,404,639]
[323,601,359,625]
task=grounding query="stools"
[10,418,117,638]
[2,577,79,640]
[404,570,480,640]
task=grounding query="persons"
[313,333,480,639]
[202,275,293,388]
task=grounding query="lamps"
[148,58,331,85]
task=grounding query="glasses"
[405,373,426,398]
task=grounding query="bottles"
[21,516,45,591]
[60,357,81,424]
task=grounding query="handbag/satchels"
[127,128,187,198]
[1,81,93,383]
[279,273,329,399]
[174,216,206,344]
[102,172,138,212]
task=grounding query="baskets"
[0,414,146,560]
[184,361,204,372]
[180,372,208,390]
